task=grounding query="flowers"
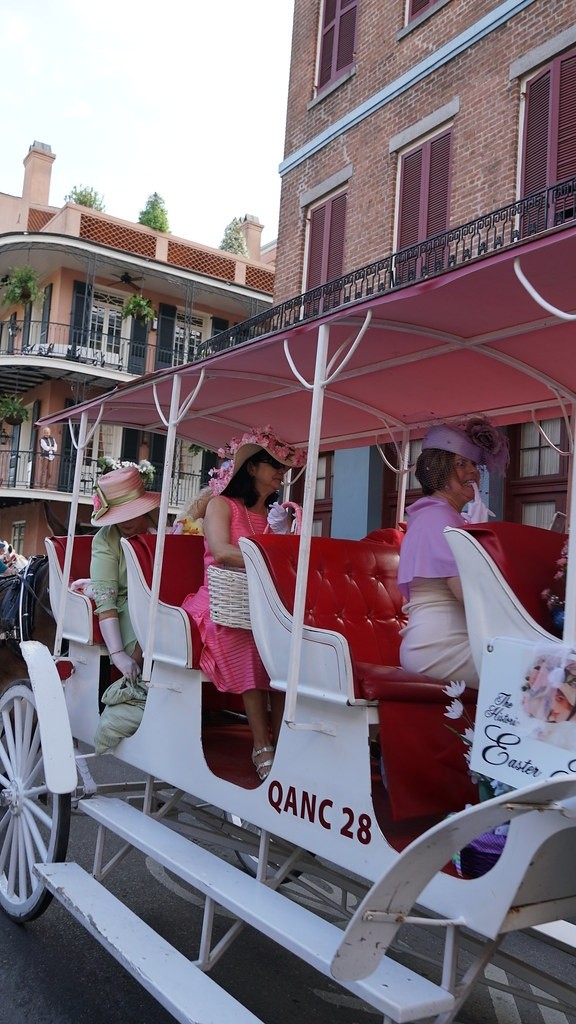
[207,424,307,495]
[456,414,511,476]
[88,486,113,520]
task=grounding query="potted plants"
[120,293,155,323]
[0,265,47,311]
[0,393,28,426]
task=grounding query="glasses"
[252,457,286,470]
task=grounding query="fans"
[107,273,145,291]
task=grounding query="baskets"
[205,501,302,630]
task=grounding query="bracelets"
[110,650,125,656]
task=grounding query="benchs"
[238,521,576,817]
[120,534,275,752]
[41,536,114,709]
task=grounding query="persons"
[396,425,495,804]
[174,451,238,527]
[181,441,294,780]
[89,467,162,684]
[39,428,58,490]
[0,543,12,574]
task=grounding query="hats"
[208,424,308,496]
[421,414,510,473]
[90,466,161,526]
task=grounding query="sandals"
[252,746,274,781]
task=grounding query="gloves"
[462,482,496,524]
[266,500,292,534]
[99,616,142,683]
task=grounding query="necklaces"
[243,503,268,535]
